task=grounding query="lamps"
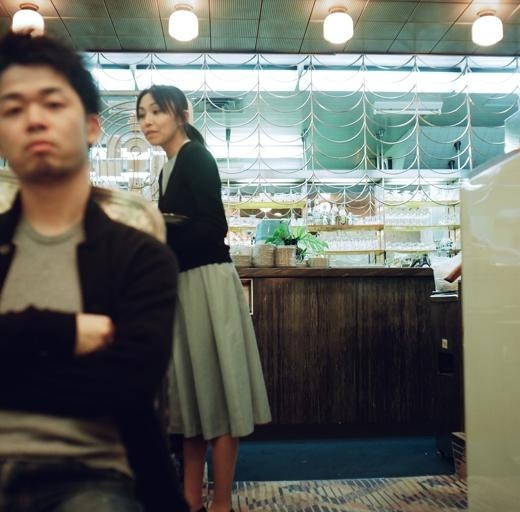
[471,10,503,46]
[372,102,443,116]
[323,6,354,45]
[168,4,199,42]
[12,3,45,37]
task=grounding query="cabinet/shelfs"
[220,179,461,266]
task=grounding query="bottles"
[226,200,457,267]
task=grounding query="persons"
[132,85,273,512]
[0,27,192,510]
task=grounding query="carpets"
[203,433,466,512]
